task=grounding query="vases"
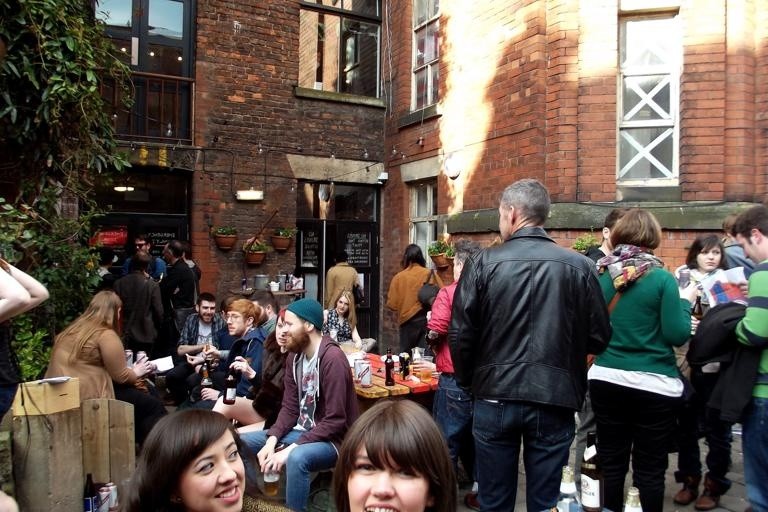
[241,249,266,266]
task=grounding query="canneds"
[242,278,248,291]
[138,351,147,364]
[400,353,410,380]
[98,482,119,512]
[125,350,133,369]
[354,360,371,387]
[292,277,303,291]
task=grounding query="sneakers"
[464,494,480,511]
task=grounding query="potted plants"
[568,231,600,255]
[269,226,299,251]
[212,225,239,250]
[428,240,449,269]
[443,247,455,269]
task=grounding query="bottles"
[555,429,642,511]
[199,367,213,388]
[205,343,217,373]
[82,473,99,512]
[384,347,394,386]
[284,272,291,291]
[222,367,238,405]
[689,294,702,336]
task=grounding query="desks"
[347,352,440,415]
[228,288,307,302]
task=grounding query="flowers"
[243,235,269,253]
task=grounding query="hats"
[288,298,323,330]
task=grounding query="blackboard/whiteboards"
[296,224,376,273]
[142,230,180,259]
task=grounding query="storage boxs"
[9,375,83,419]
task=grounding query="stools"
[304,467,333,504]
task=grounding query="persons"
[0,259,49,508]
[45,253,458,511]
[387,180,767,511]
[93,236,202,360]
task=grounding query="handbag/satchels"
[172,307,195,332]
[418,283,439,305]
[353,285,362,304]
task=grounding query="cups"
[411,347,433,382]
[263,468,280,497]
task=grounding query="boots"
[673,471,702,505]
[695,472,731,511]
